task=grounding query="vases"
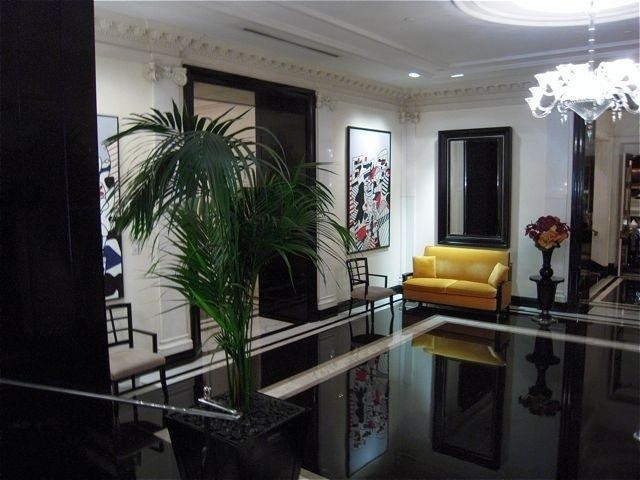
[535,362,550,388]
[540,250,554,277]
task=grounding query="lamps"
[522,0,640,123]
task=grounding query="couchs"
[411,334,507,367]
[402,246,513,323]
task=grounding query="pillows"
[411,255,437,278]
[488,261,510,288]
[411,335,434,349]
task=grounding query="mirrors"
[438,126,513,247]
[431,355,503,471]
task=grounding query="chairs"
[109,382,166,469]
[345,257,396,337]
[105,301,168,419]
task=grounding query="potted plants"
[105,103,358,480]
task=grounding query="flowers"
[519,385,561,417]
[524,215,570,251]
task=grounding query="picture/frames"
[345,124,391,255]
[98,114,124,299]
[346,350,389,476]
